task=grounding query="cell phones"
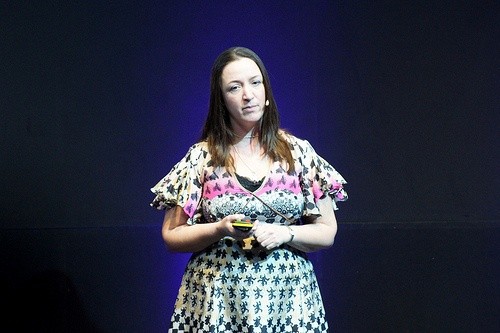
[230,221,253,231]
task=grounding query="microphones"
[266,99,269,106]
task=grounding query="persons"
[150,45,349,333]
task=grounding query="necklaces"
[232,132,264,140]
[231,139,269,176]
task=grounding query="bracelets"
[285,225,295,244]
